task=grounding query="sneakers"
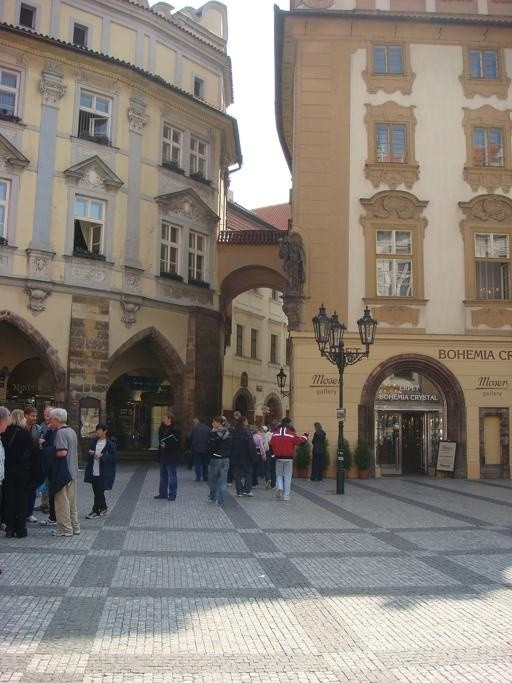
[1,505,81,538]
[265,479,289,501]
[226,482,260,496]
[154,495,176,501]
[85,509,107,519]
[207,495,224,508]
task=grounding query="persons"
[0,406,81,538]
[185,407,326,507]
[152,410,180,500]
[83,424,117,519]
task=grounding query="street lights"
[312,300,377,495]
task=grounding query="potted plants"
[293,439,311,478]
[344,439,352,479]
[355,436,372,479]
[322,439,330,478]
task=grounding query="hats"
[262,426,268,431]
